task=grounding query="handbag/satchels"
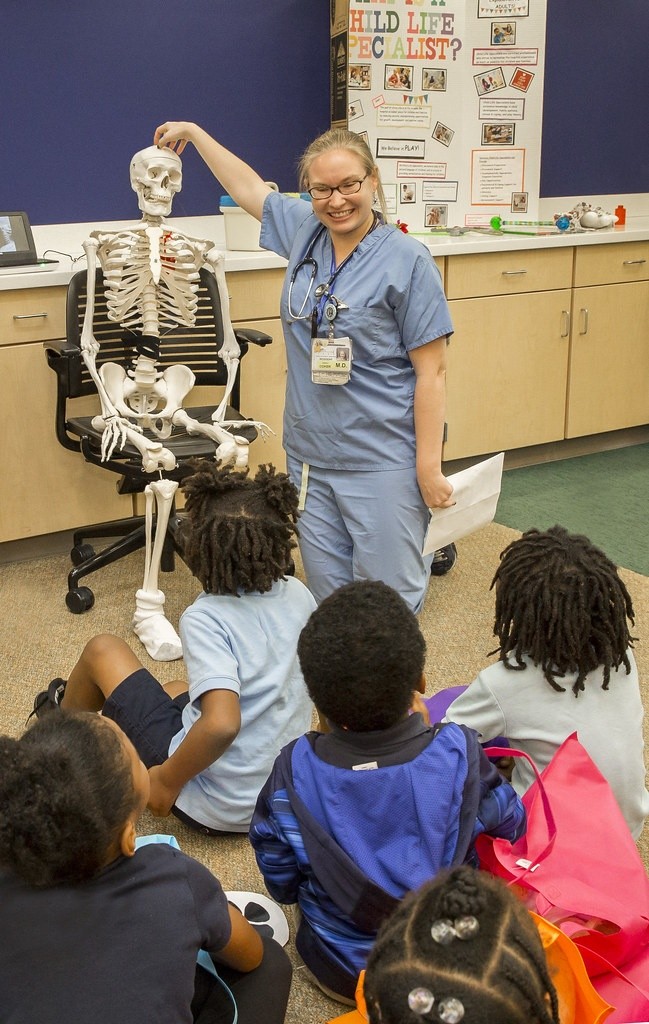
[473,731,648,1024]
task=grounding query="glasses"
[308,172,370,200]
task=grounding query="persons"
[250,579,526,1009]
[427,207,444,225]
[154,120,456,614]
[350,106,356,116]
[337,351,346,361]
[484,125,511,143]
[0,708,292,1024]
[362,866,560,1024]
[494,24,513,43]
[351,66,369,87]
[410,525,649,844]
[482,77,497,91]
[521,197,525,203]
[403,185,414,200]
[34,458,317,836]
[388,68,410,88]
[436,125,447,141]
[424,71,445,89]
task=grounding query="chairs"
[39,261,276,618]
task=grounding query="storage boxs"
[219,192,313,251]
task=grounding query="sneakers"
[24,677,70,726]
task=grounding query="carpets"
[491,442,649,576]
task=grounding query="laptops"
[0,211,60,268]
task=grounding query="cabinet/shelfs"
[442,239,649,479]
[0,283,134,544]
[133,256,445,518]
